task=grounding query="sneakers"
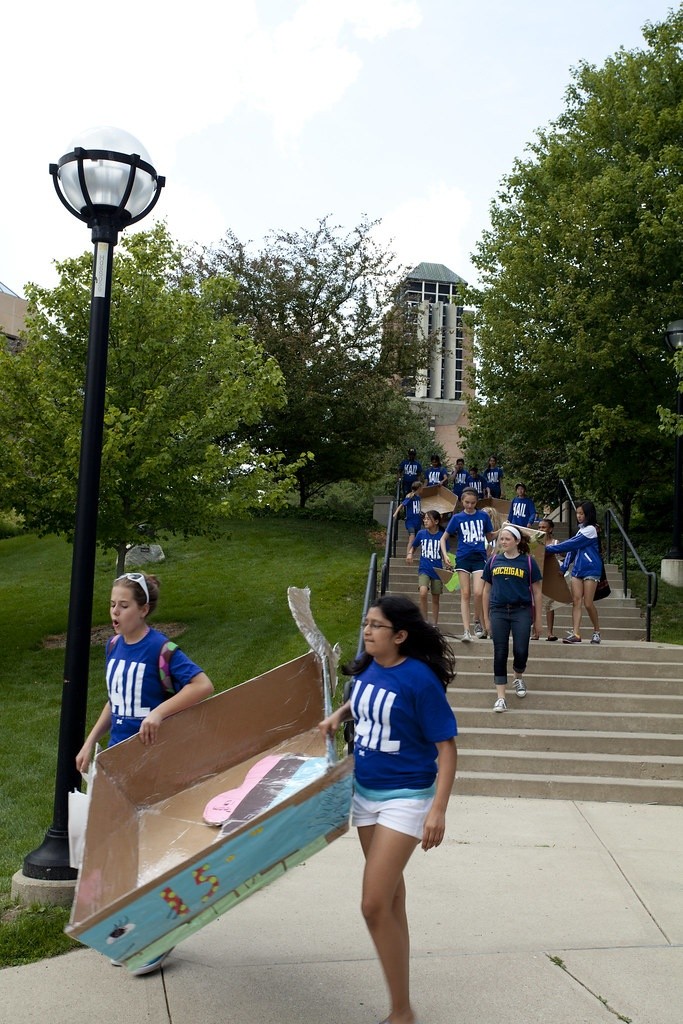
[473,616,482,633]
[591,631,602,644]
[493,698,508,712]
[513,678,527,697]
[134,947,175,976]
[563,630,582,643]
[111,960,119,966]
[461,631,473,641]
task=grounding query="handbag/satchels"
[68,762,96,871]
[594,577,611,601]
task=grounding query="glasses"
[361,622,393,630]
[114,572,150,604]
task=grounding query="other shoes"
[532,635,539,640]
[548,635,558,641]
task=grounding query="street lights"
[662,320,683,559]
[23,122,166,880]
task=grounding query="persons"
[506,482,536,528]
[545,500,603,644]
[440,487,510,642]
[422,455,448,520]
[481,525,543,712]
[76,570,214,975]
[466,467,492,501]
[483,456,503,498]
[481,507,500,639]
[531,519,567,641]
[393,482,423,563]
[399,448,422,519]
[451,459,470,515]
[406,511,450,631]
[318,595,458,1024]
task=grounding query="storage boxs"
[475,496,512,526]
[420,484,459,523]
[62,582,356,972]
[495,521,574,614]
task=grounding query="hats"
[514,482,527,490]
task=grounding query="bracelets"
[408,552,413,556]
